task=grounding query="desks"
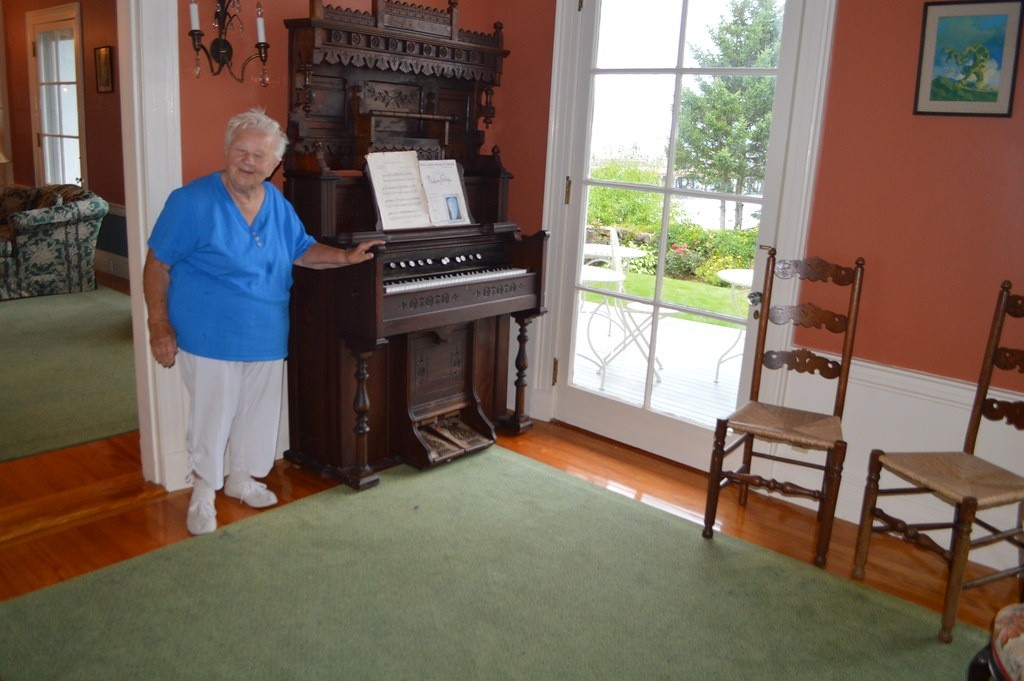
[583,244,647,340]
[576,265,626,391]
[713,268,754,383]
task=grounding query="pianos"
[270,0,551,490]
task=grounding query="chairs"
[702,243,865,568]
[596,227,679,383]
[851,279,1024,644]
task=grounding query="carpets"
[1,281,139,462]
[0,441,992,680]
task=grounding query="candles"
[189,0,200,30]
[256,1,266,42]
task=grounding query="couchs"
[0,183,109,301]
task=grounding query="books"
[364,151,471,231]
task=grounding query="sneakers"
[224,478,277,507]
[186,497,217,534]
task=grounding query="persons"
[142,106,385,536]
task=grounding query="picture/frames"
[94,46,114,93]
[913,0,1024,117]
